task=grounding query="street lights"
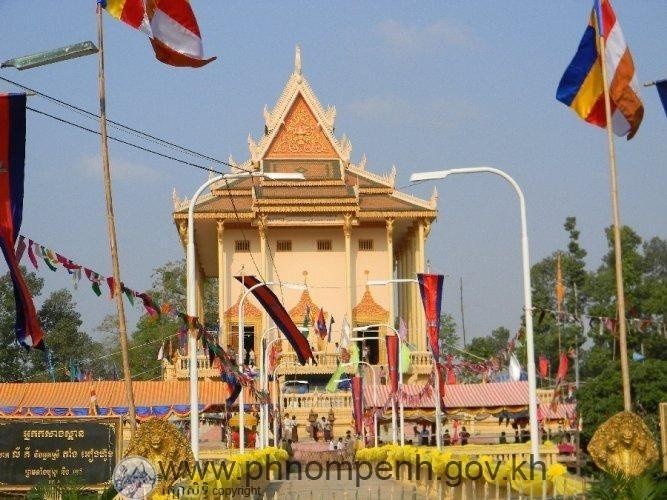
[238,280,308,453]
[341,361,379,449]
[272,361,301,447]
[186,170,305,462]
[264,336,288,447]
[409,165,541,469]
[364,278,444,449]
[258,324,303,450]
[352,336,399,446]
[280,380,307,453]
[352,324,406,448]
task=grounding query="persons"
[278,412,570,460]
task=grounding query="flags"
[654,77,667,114]
[97,0,216,67]
[556,0,645,140]
[0,91,666,415]
